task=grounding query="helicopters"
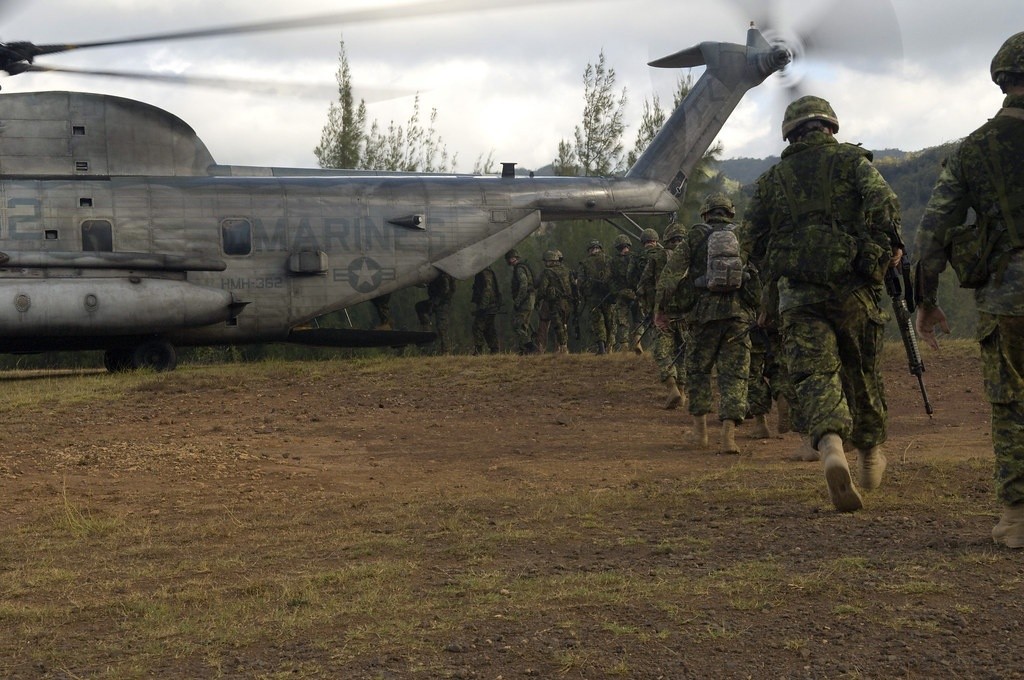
[0,0,906,373]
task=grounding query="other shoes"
[491,347,499,356]
[368,318,393,330]
[607,344,612,354]
[621,344,628,355]
[595,342,605,355]
[635,335,643,355]
[472,346,483,357]
[553,346,569,354]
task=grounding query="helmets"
[586,239,603,250]
[543,248,563,261]
[640,228,659,242]
[664,223,688,240]
[700,192,736,216]
[990,31,1024,85]
[782,95,839,141]
[614,234,632,248]
[505,249,522,259]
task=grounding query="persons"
[636,193,821,463]
[916,30,1024,549]
[371,293,393,330]
[536,248,581,356]
[471,266,506,358]
[739,96,907,513]
[578,229,663,357]
[415,272,457,357]
[505,249,539,356]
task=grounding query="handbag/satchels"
[946,223,988,289]
[670,278,698,315]
[545,287,556,300]
[772,226,857,284]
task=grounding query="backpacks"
[691,223,743,292]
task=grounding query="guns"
[631,317,653,343]
[884,264,933,415]
[665,341,685,371]
[590,292,613,312]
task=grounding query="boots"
[818,434,863,511]
[991,506,1024,548]
[677,383,686,406]
[777,393,792,433]
[789,433,820,461]
[664,377,681,409]
[859,445,887,488]
[684,416,708,446]
[747,416,769,438]
[721,420,741,453]
